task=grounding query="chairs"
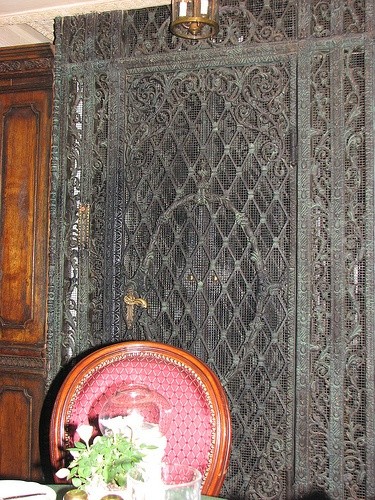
[48,340,234,496]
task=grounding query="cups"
[125,461,201,500]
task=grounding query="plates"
[0,480,56,500]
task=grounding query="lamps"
[170,0,219,39]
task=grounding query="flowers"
[53,412,160,499]
